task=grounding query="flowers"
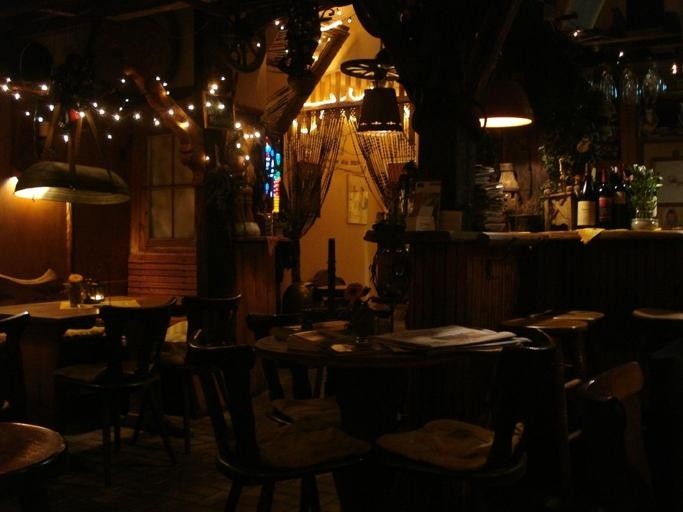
[622,161,661,219]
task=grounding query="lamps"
[12,104,131,205]
[357,39,405,139]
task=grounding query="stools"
[502,306,683,512]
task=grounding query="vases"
[630,196,659,231]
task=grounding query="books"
[471,163,507,231]
[368,323,533,356]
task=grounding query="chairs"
[155,295,244,455]
[187,342,374,512]
[1,310,29,420]
[245,312,297,338]
[51,299,174,484]
[378,345,551,510]
[302,309,353,331]
[0,270,59,302]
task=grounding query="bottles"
[597,169,614,228]
[614,162,631,228]
[575,162,597,229]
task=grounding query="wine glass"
[583,57,668,105]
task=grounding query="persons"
[427,191,440,219]
[665,208,680,227]
